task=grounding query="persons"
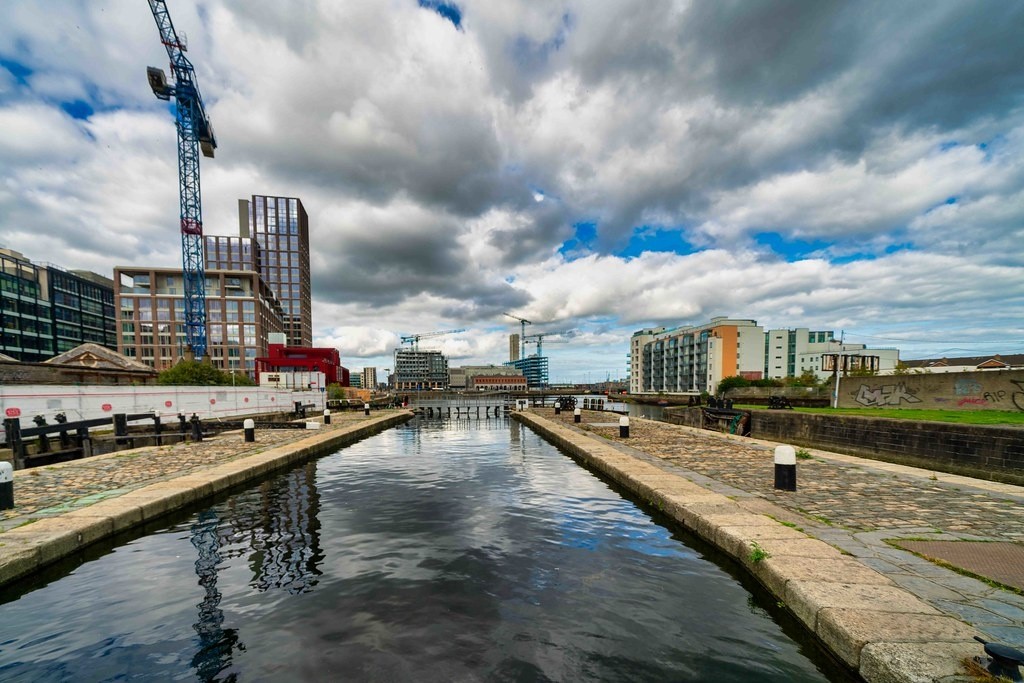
[688,395,701,407]
[706,395,733,409]
[393,393,402,407]
[404,393,408,404]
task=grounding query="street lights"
[829,338,843,409]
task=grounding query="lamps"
[0,461,14,483]
[243,419,255,428]
[620,416,629,426]
[773,445,796,466]
[324,409,330,416]
[555,402,561,408]
[573,408,581,415]
[365,403,370,409]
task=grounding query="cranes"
[400,328,466,350]
[502,312,532,360]
[521,330,572,340]
[524,340,569,357]
[401,333,440,350]
[143,0,216,365]
